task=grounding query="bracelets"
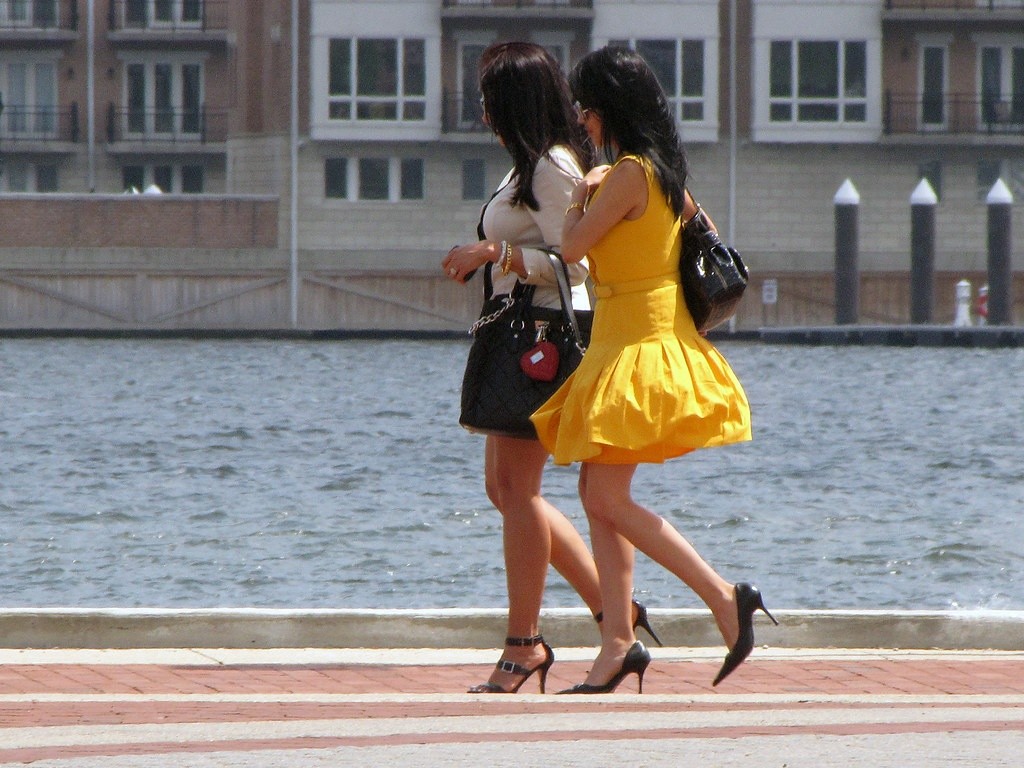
[502,244,512,275]
[495,240,506,267]
[566,203,584,216]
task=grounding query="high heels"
[712,581,780,687]
[554,640,651,695]
[593,596,663,647]
[465,635,555,693]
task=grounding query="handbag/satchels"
[458,250,597,440]
[680,186,749,332]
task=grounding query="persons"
[441,42,663,694]
[530,47,778,695]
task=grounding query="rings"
[451,269,457,275]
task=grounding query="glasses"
[572,101,596,121]
